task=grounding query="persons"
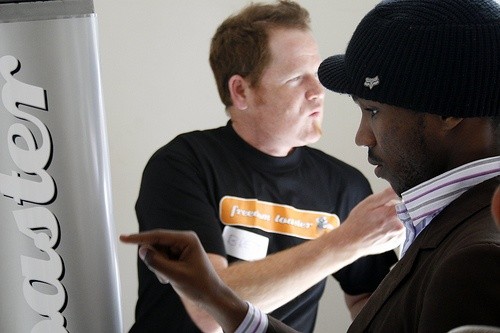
[119,0,499,332]
[129,1,398,333]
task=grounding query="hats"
[318,0,500,117]
